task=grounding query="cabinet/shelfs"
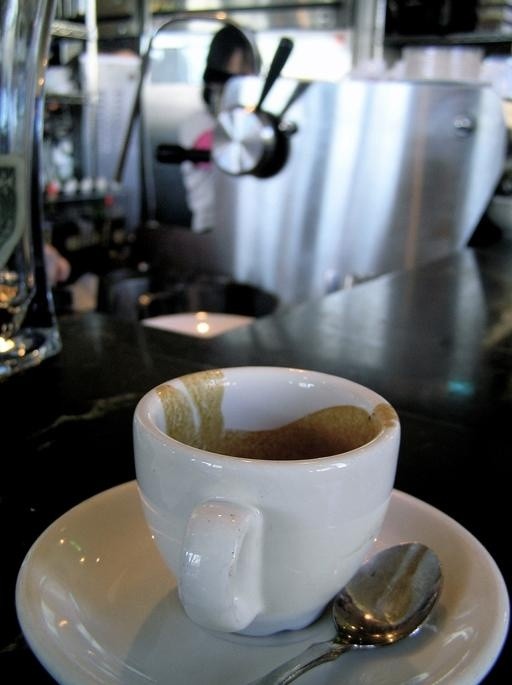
[0,1,99,195]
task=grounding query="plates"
[16,477,509,685]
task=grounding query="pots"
[207,39,297,178]
[201,27,260,116]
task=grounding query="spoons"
[253,543,441,685]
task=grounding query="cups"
[129,365,405,639]
